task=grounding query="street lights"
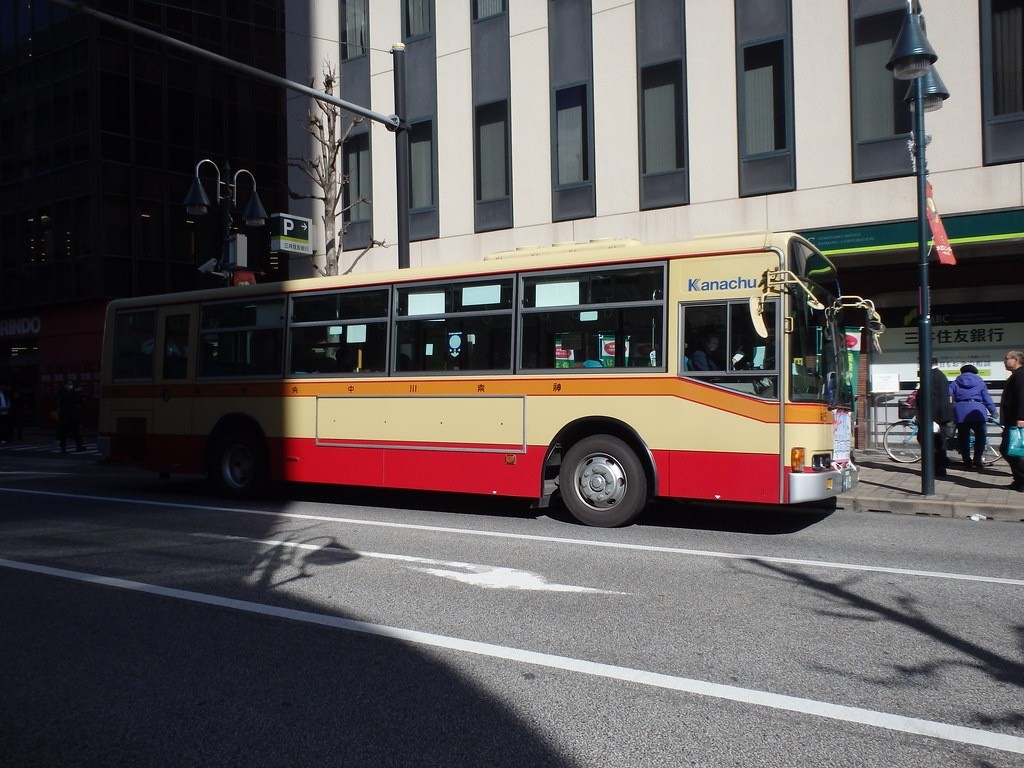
[884,0,952,494]
[182,159,272,287]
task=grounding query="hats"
[961,365,978,375]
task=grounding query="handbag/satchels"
[1007,426,1024,458]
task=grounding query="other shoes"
[973,461,981,466]
[1001,482,1024,492]
[965,460,974,469]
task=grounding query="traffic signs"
[270,212,313,259]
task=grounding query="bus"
[94,214,887,527]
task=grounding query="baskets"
[898,399,915,419]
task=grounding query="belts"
[962,399,982,402]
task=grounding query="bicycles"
[881,397,1007,467]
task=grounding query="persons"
[827,370,851,404]
[56,379,87,455]
[0,384,27,445]
[203,342,230,378]
[399,351,430,371]
[915,356,952,453]
[335,346,371,372]
[142,336,181,380]
[947,365,999,470]
[686,327,745,371]
[999,350,1024,493]
